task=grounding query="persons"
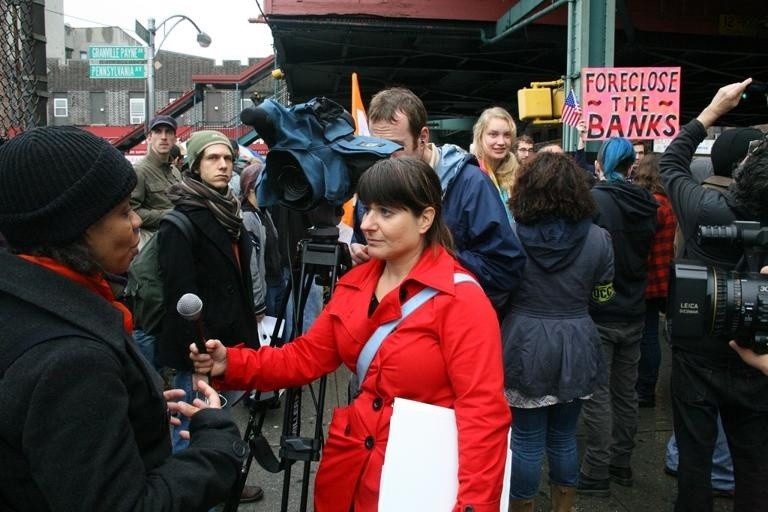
[499,138,678,512]
[468,107,562,210]
[1,128,251,512]
[189,155,511,512]
[124,114,278,502]
[350,89,527,304]
[658,78,768,512]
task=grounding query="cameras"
[742,84,768,100]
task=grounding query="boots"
[550,484,577,512]
[509,498,535,512]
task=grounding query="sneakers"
[577,465,633,496]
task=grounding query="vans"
[643,136,714,182]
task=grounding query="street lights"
[147,15,212,138]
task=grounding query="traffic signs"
[86,45,146,61]
[133,18,150,47]
[87,64,146,80]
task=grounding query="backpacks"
[128,214,196,336]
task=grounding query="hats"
[149,115,176,131]
[1,126,136,249]
[187,130,235,172]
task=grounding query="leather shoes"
[239,487,265,503]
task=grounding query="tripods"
[224,240,344,512]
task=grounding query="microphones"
[177,293,207,354]
